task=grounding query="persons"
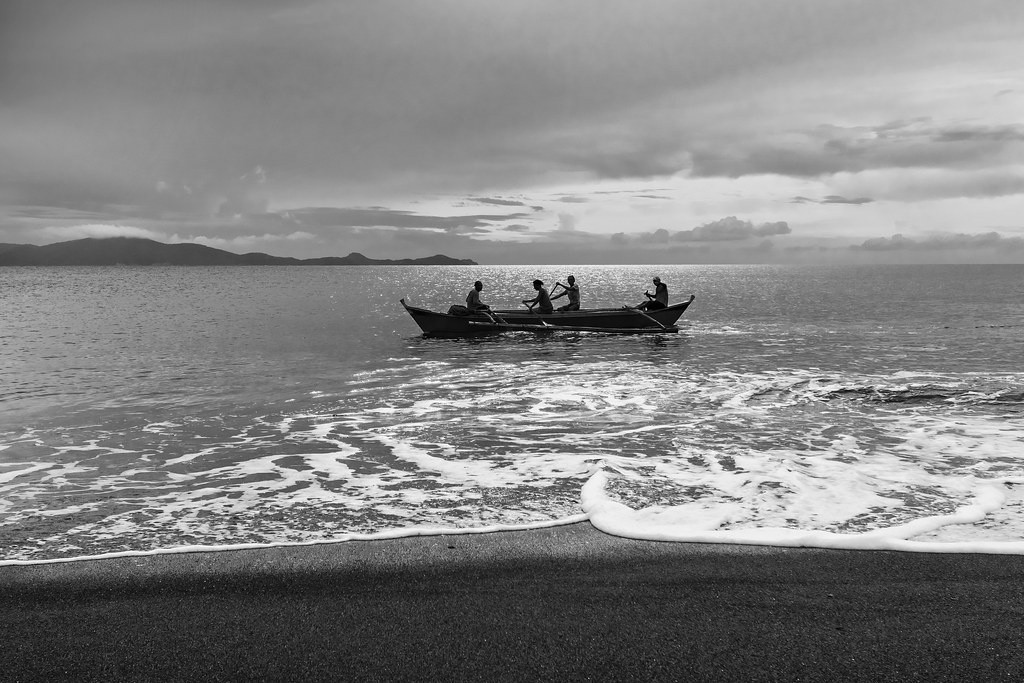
[466,281,489,312]
[522,280,553,312]
[635,276,668,310]
[550,275,580,311]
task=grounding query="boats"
[399,294,698,340]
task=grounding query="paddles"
[549,283,558,297]
[487,306,508,323]
[523,302,548,326]
[644,290,653,301]
